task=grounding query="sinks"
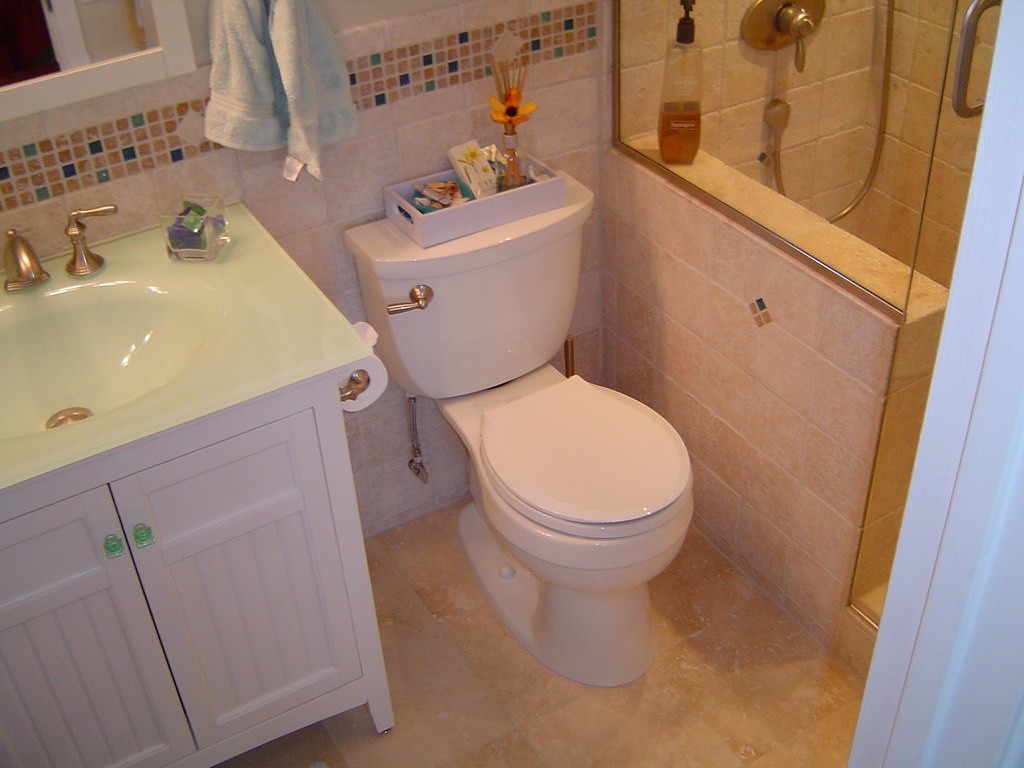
[0,269,226,452]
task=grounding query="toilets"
[341,164,697,691]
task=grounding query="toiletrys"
[657,0,702,166]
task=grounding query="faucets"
[4,233,49,290]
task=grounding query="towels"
[203,0,362,184]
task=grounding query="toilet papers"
[338,318,389,413]
